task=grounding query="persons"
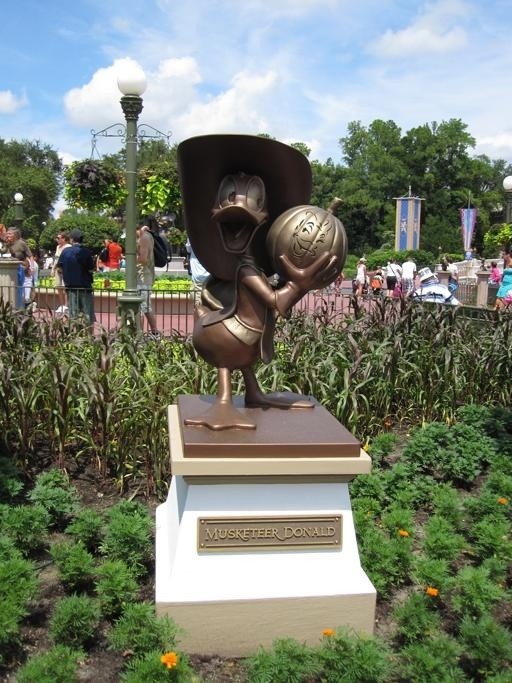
[334,247,511,310]
[0,214,210,338]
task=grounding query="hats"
[67,230,83,239]
[419,267,435,283]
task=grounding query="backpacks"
[147,230,168,267]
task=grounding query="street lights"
[502,172,511,222]
[12,191,25,240]
[89,70,171,344]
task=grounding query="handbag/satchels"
[100,247,108,262]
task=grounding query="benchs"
[350,273,417,300]
